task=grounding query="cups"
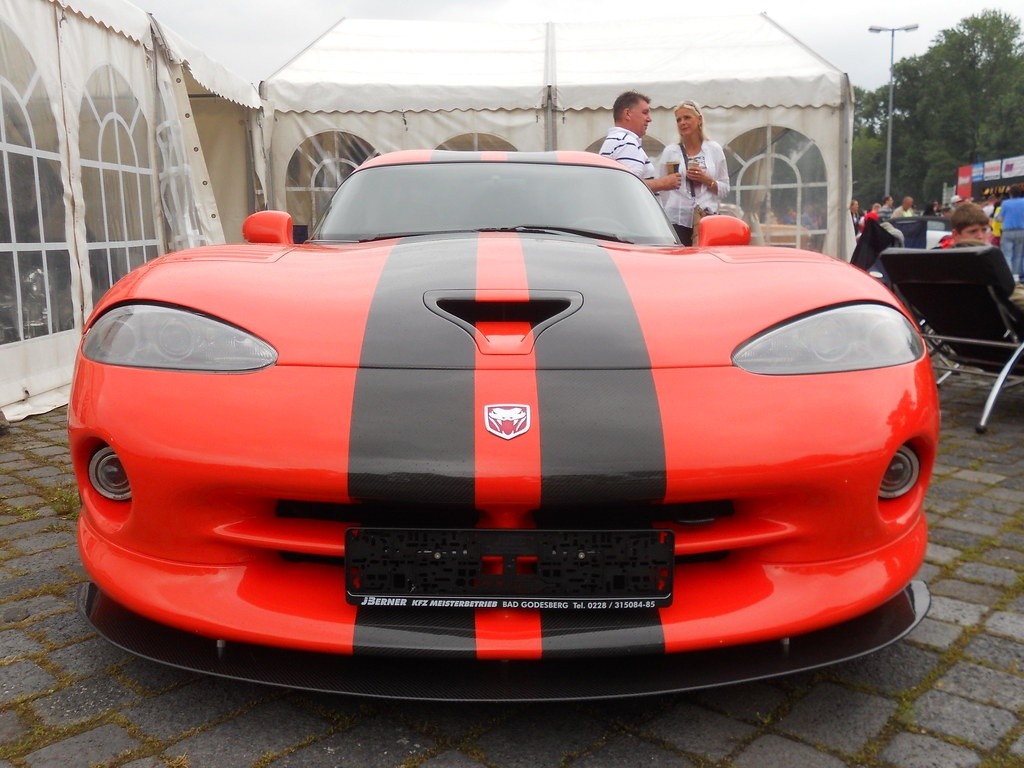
[665,161,679,175]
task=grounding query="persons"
[659,100,730,247]
[851,196,914,234]
[930,204,990,249]
[598,91,681,208]
[997,184,1024,281]
[769,203,827,229]
[921,195,1003,247]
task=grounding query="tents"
[259,12,857,263]
[0,0,274,421]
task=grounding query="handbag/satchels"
[692,205,717,245]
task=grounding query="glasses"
[684,100,700,114]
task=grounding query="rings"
[695,171,698,173]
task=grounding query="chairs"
[760,224,810,249]
[880,247,1024,434]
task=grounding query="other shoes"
[1013,274,1020,282]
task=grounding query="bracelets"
[708,182,714,189]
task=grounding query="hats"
[950,195,962,203]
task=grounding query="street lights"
[868,24,920,196]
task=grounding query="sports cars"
[64,148,938,704]
